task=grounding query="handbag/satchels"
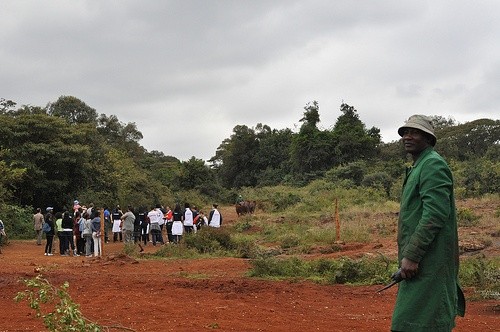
[83,229,92,237]
[97,232,101,237]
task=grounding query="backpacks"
[42,223,51,232]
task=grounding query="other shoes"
[35,239,181,257]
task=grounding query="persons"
[32,198,223,259]
[386,113,461,332]
[0,219,6,253]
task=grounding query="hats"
[398,114,437,147]
[43,192,244,212]
